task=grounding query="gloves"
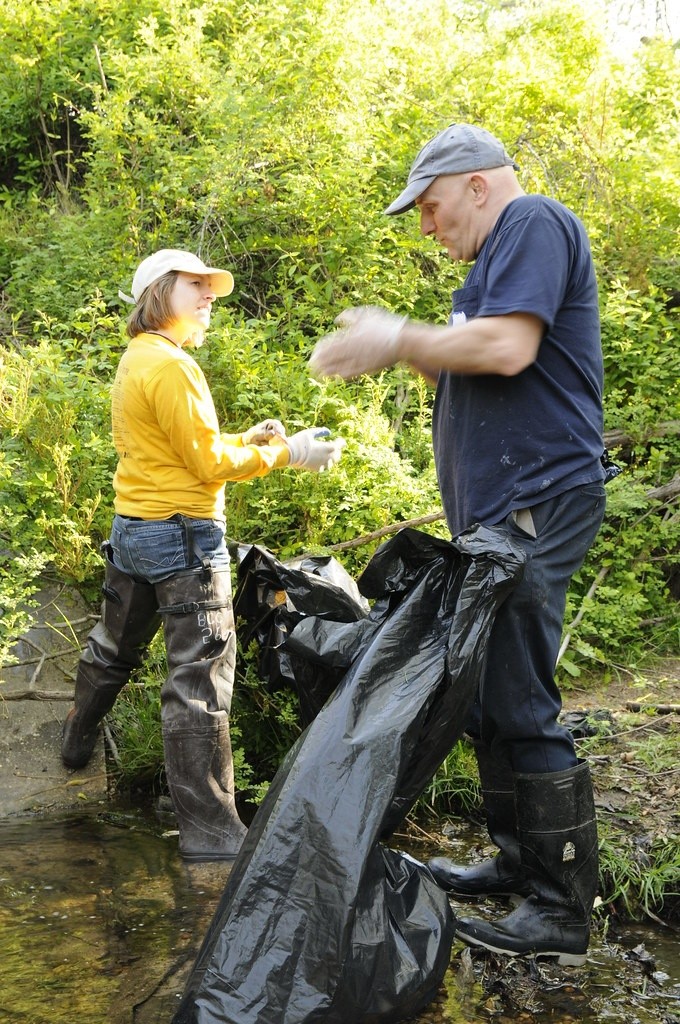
[285,424,347,475]
[305,303,408,385]
[242,417,288,448]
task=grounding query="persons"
[60,249,339,862]
[309,125,607,967]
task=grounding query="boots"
[162,719,256,861]
[427,749,526,901]
[454,757,601,968]
[58,654,132,772]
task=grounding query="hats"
[117,247,235,304]
[383,122,521,216]
